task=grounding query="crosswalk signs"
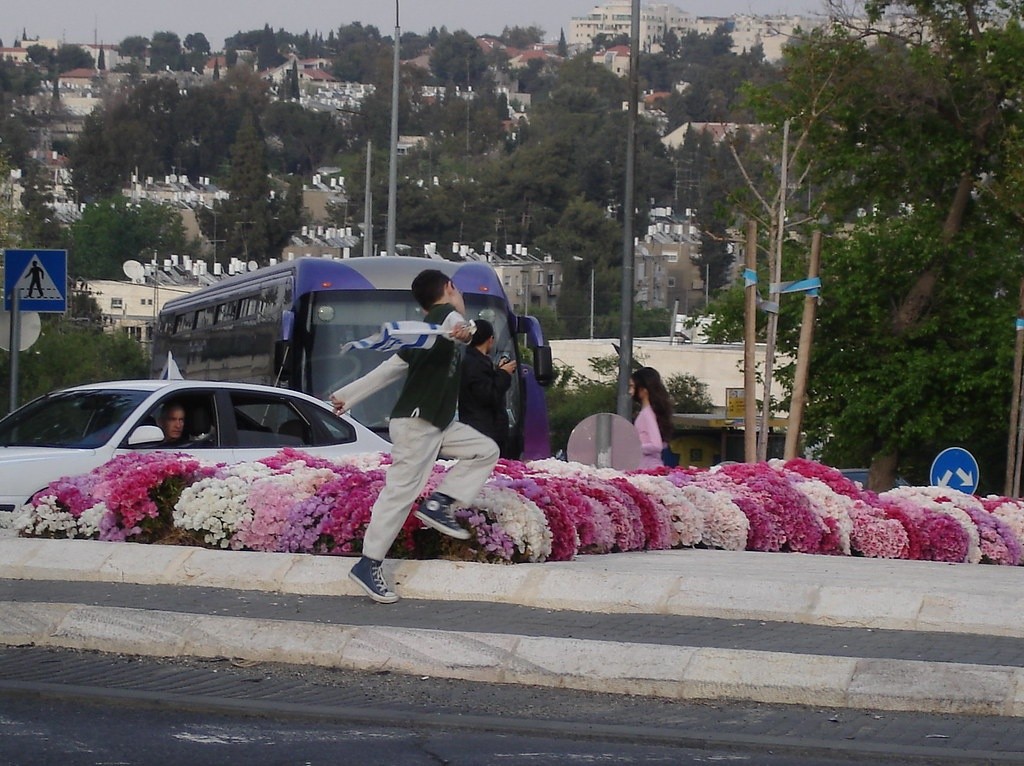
[2,248,68,315]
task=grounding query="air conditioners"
[544,257,552,262]
[693,280,704,289]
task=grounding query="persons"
[154,400,185,448]
[626,367,672,473]
[331,269,500,604]
[458,320,518,459]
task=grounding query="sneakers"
[348,555,400,604]
[413,491,472,540]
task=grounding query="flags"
[338,320,445,352]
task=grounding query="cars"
[838,468,911,494]
[1,378,395,535]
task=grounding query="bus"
[148,254,556,461]
